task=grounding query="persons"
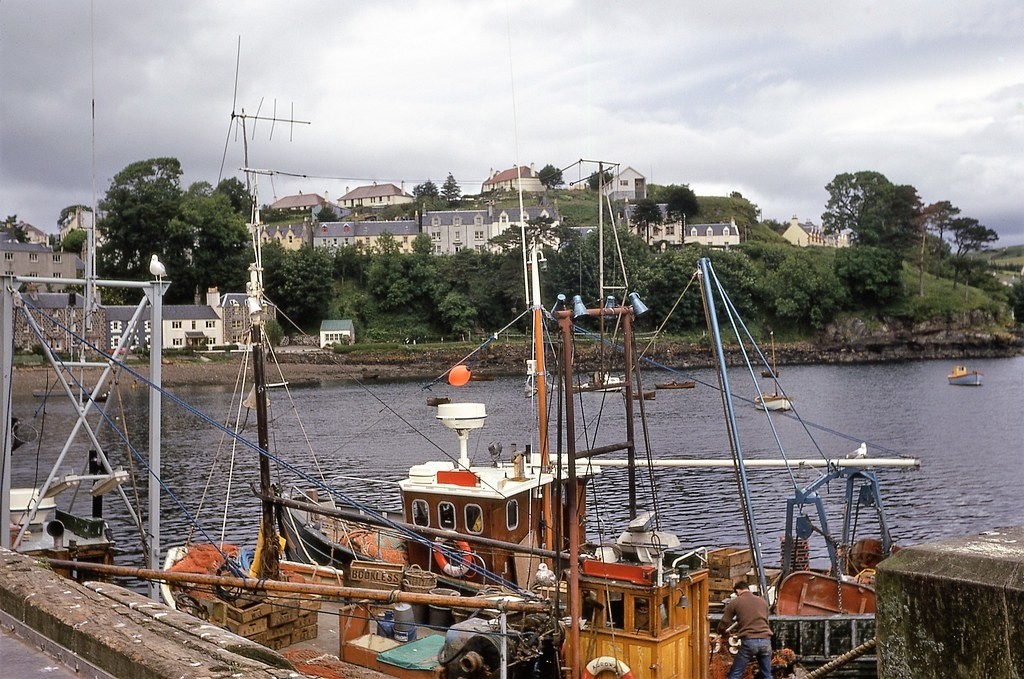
[717,581,774,679]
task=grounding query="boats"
[947,366,984,385]
[581,372,623,393]
[761,368,780,379]
[653,382,696,389]
[621,390,656,401]
[3,36,924,679]
[426,397,451,405]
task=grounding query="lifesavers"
[581,656,633,679]
[433,530,472,577]
[561,639,566,664]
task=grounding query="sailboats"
[755,331,793,413]
